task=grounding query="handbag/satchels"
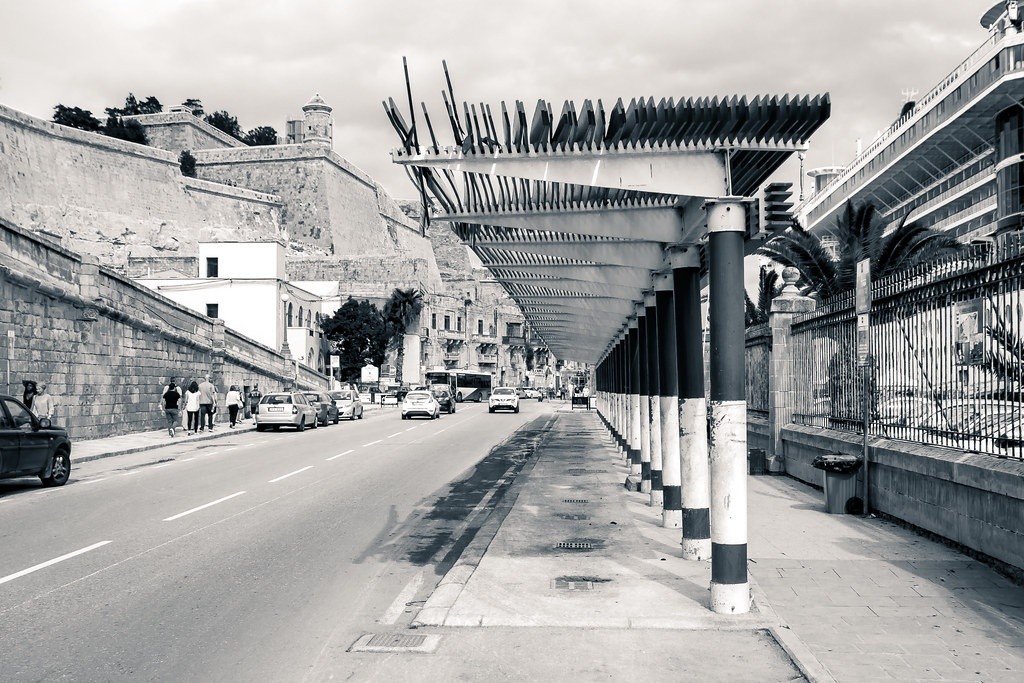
[237,398,245,410]
[207,402,216,415]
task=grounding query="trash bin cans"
[821,455,863,514]
[747,447,767,475]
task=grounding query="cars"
[0,396,73,487]
[811,394,1024,458]
[516,386,555,399]
[327,390,363,420]
[302,391,339,426]
[430,389,456,413]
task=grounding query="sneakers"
[169,428,174,438]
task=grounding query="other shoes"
[209,428,213,432]
[230,422,233,428]
[188,431,191,436]
[252,421,256,425]
[233,427,235,428]
[200,430,205,432]
[195,432,200,435]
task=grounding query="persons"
[226,384,245,429]
[248,382,263,425]
[161,376,183,417]
[161,383,182,437]
[22,379,54,419]
[560,384,566,399]
[182,380,201,436]
[198,374,218,432]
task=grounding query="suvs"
[401,391,441,420]
[256,392,319,432]
[489,386,519,413]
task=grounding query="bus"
[424,366,491,402]
